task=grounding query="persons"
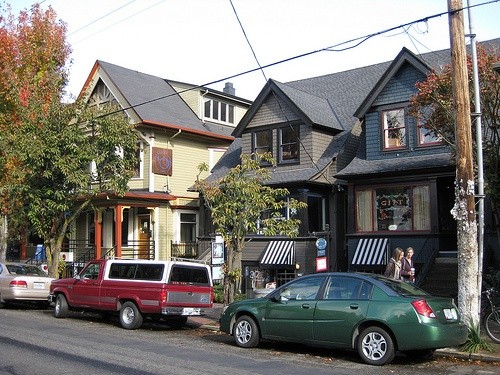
[384,247,404,280]
[400,246,416,282]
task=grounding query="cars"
[217,271,469,366]
[0,262,56,305]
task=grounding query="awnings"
[260,240,295,265]
[351,237,389,265]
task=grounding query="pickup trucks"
[49,259,214,330]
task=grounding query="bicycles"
[481,287,500,344]
